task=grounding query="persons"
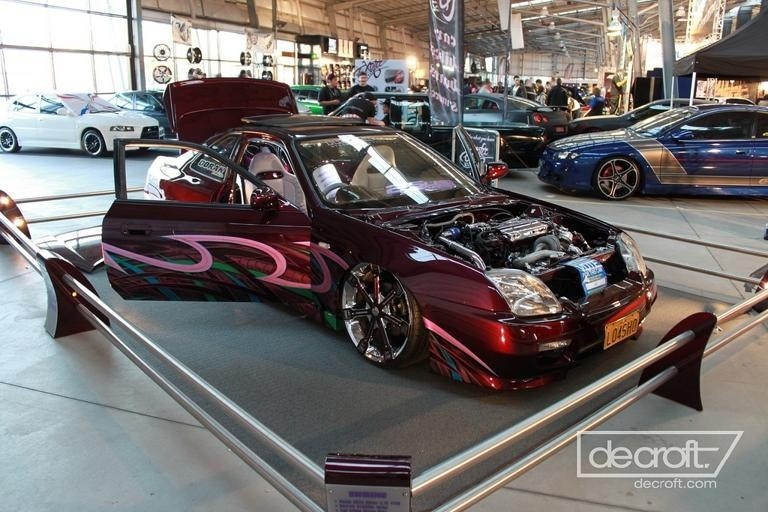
[461,75,605,118]
[318,72,340,115]
[340,94,379,122]
[376,99,394,126]
[239,69,248,78]
[348,74,375,99]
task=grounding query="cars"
[0,90,177,158]
[287,84,768,201]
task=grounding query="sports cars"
[102,77,658,393]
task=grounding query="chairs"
[246,145,402,219]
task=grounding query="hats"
[379,99,390,105]
[326,73,339,80]
